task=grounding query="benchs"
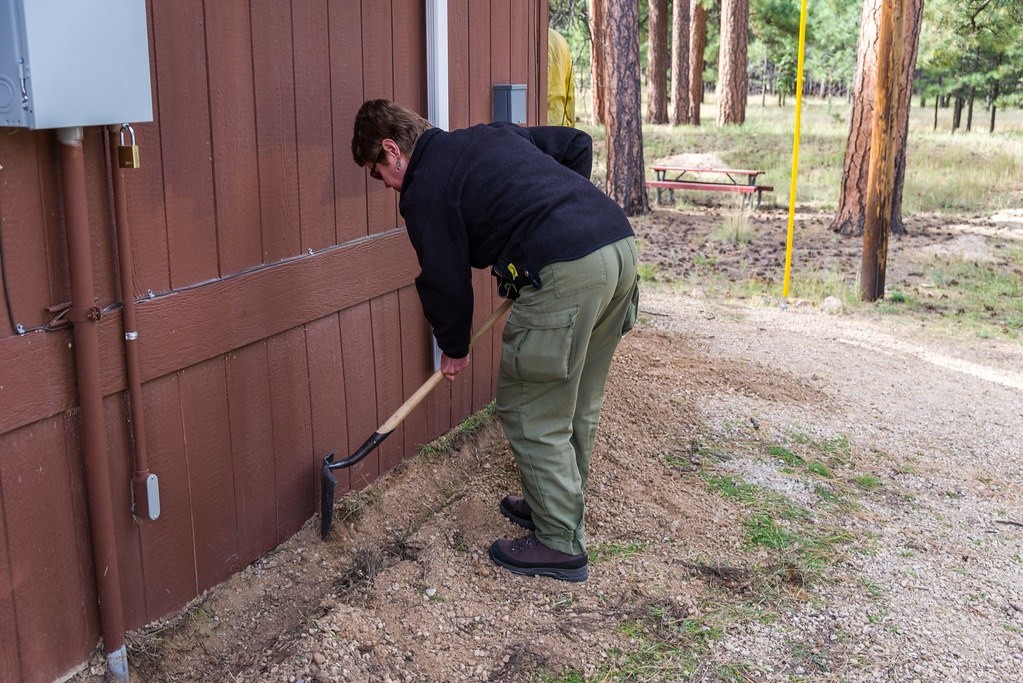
[644,181,773,193]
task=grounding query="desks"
[651,166,766,212]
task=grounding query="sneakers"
[500,495,537,530]
[489,531,589,582]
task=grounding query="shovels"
[319,289,519,542]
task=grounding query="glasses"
[370,146,384,180]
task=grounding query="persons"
[351,99,640,582]
[545,28,575,126]
